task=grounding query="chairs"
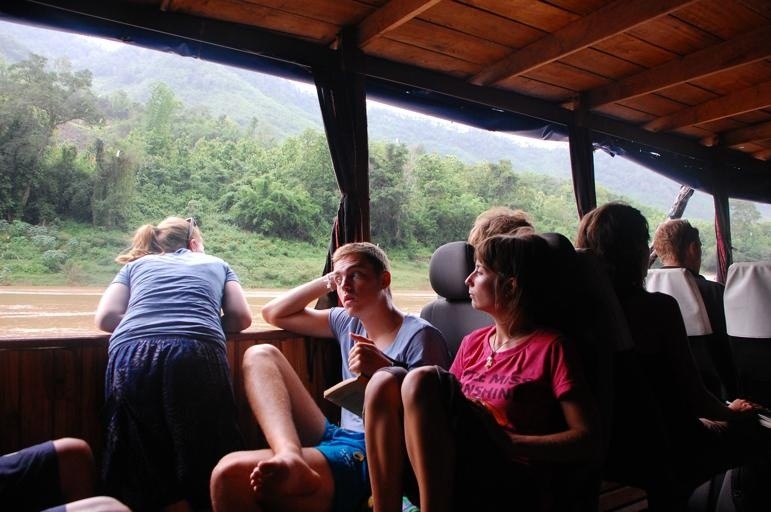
[639,263,722,394]
[720,258,769,409]
[528,232,742,509]
[418,237,496,364]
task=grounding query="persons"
[0,437,133,512]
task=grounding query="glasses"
[185,216,198,244]
